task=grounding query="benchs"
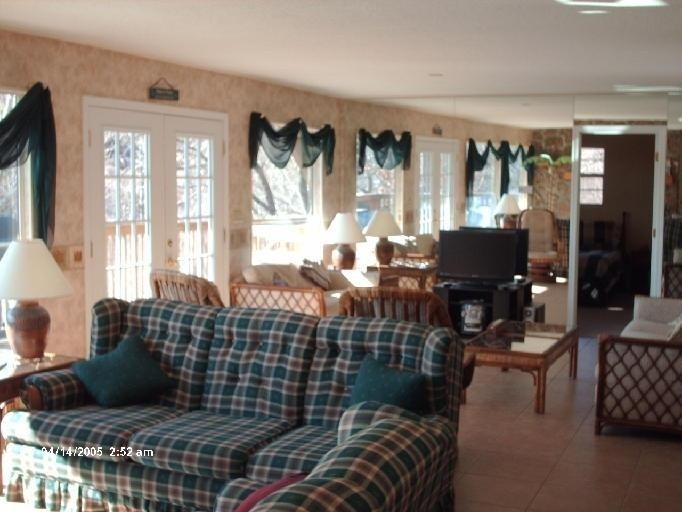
[591,291,681,440]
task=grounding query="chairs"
[222,401,450,512]
[579,210,629,307]
[146,265,475,395]
[514,208,566,283]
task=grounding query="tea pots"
[340,94,572,330]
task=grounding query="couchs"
[0,296,465,512]
[366,233,446,289]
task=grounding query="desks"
[0,346,84,405]
[461,319,583,414]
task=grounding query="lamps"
[323,211,368,270]
[579,210,629,307]
[0,236,78,358]
[514,208,566,283]
[222,401,450,512]
[495,193,523,228]
[363,208,403,265]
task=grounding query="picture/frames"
[0,346,84,405]
[461,319,583,414]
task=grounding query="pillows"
[345,352,425,413]
[301,258,333,291]
[68,333,170,409]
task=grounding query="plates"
[517,277,534,306]
[433,279,524,343]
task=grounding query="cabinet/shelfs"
[433,279,524,343]
[517,277,534,306]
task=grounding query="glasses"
[363,208,403,265]
[495,193,523,228]
[323,211,368,270]
[0,236,78,358]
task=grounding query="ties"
[459,226,528,280]
[438,230,517,289]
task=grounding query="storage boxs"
[591,291,681,440]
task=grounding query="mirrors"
[340,94,572,330]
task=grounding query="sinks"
[0,296,465,512]
[366,233,446,289]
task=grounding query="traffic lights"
[301,258,333,291]
[345,352,425,413]
[68,333,170,409]
